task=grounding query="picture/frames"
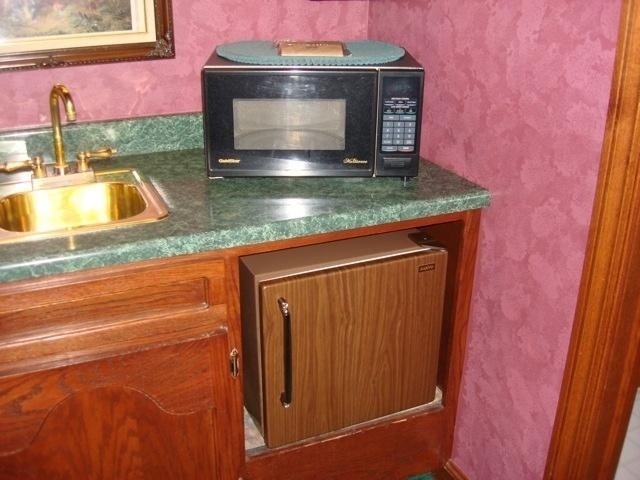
[0,0,176,72]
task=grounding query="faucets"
[49,84,77,174]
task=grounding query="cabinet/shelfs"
[0,208,482,480]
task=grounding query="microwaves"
[198,41,426,185]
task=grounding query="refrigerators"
[237,226,451,452]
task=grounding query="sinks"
[0,166,173,244]
